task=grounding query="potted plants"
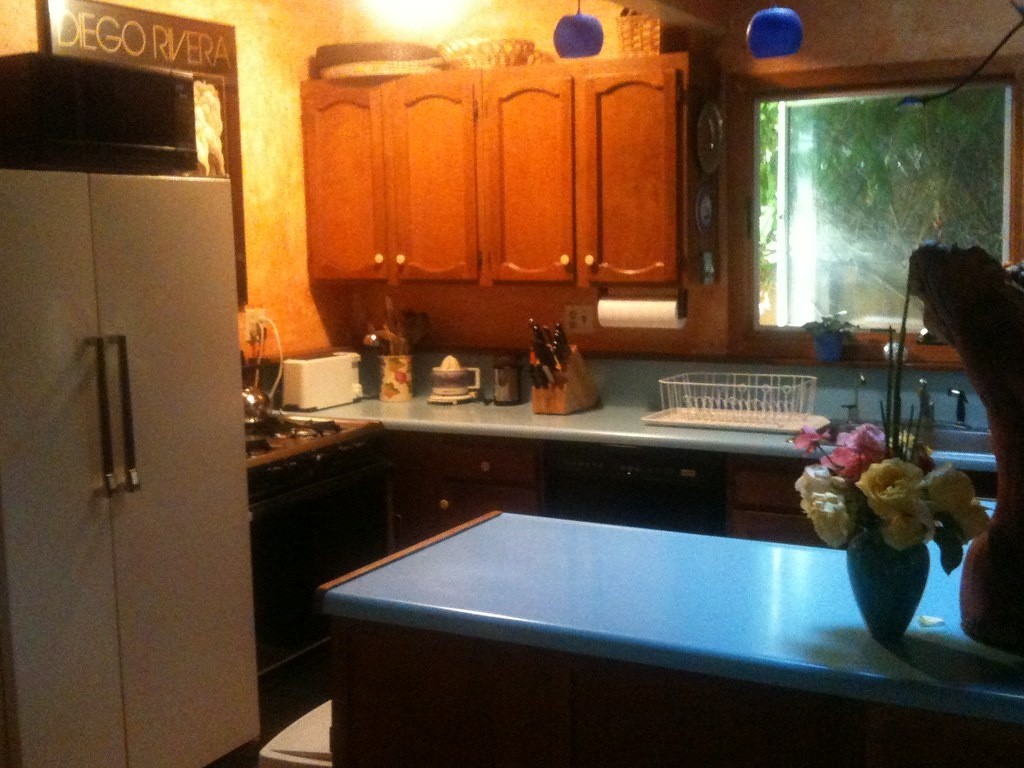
[803,315,857,365]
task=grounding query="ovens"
[247,430,396,685]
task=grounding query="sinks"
[822,427,999,459]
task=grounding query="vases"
[848,517,931,643]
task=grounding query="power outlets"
[244,308,269,344]
[566,305,595,330]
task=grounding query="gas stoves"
[243,417,386,477]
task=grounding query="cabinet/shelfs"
[249,432,1023,767]
[301,53,721,284]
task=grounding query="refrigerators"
[0,169,263,768]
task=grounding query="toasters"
[282,351,364,412]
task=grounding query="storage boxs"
[616,13,663,52]
[0,54,200,172]
[282,348,362,411]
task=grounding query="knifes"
[525,319,569,389]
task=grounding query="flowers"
[361,294,427,354]
[792,289,987,576]
[379,355,416,403]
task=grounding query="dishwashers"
[535,441,729,539]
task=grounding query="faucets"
[918,379,936,422]
[841,371,865,423]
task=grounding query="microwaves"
[0,53,197,173]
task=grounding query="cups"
[379,355,413,401]
[431,355,482,395]
[493,368,521,406]
